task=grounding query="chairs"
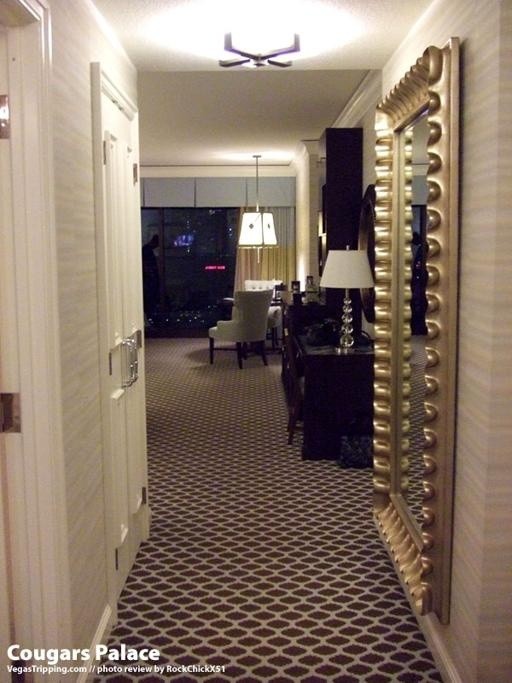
[281,324,307,444]
[206,287,275,371]
[242,277,284,350]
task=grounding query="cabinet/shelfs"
[280,290,331,384]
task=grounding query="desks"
[219,296,285,352]
[289,328,375,462]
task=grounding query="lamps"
[219,28,302,73]
[319,244,375,348]
[237,152,282,263]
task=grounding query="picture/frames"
[317,209,324,236]
